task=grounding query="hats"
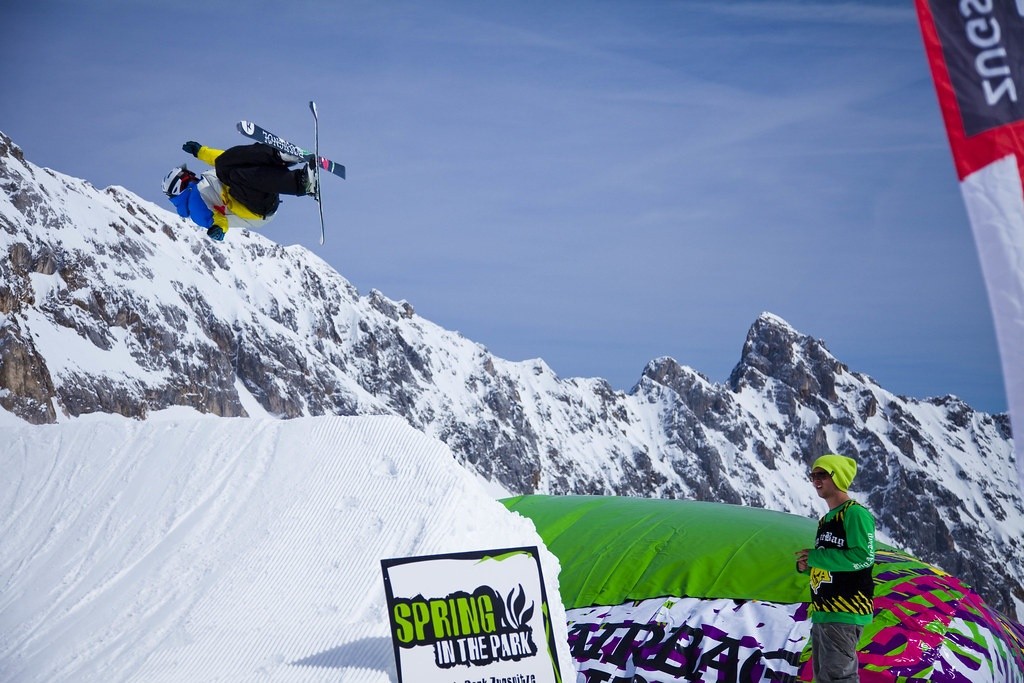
[812,455,858,492]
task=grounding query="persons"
[795,454,876,683]
[162,141,316,240]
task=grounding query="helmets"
[161,168,196,199]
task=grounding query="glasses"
[809,470,830,482]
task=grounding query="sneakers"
[294,158,317,197]
[277,149,303,167]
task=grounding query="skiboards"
[235,98,347,244]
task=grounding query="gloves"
[206,225,225,241]
[181,141,202,158]
